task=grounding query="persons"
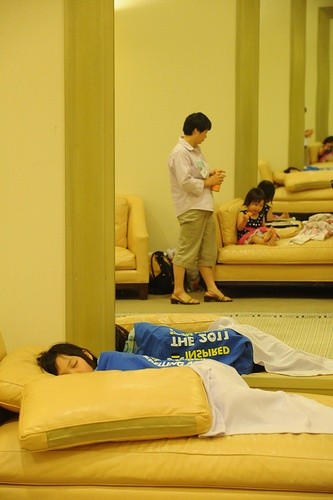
[168,112,231,304]
[258,180,288,221]
[38,341,302,432]
[282,108,333,173]
[115,322,309,375]
[236,188,277,246]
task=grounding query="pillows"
[1,349,55,413]
[19,365,213,453]
[285,170,333,192]
[115,313,219,336]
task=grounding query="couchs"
[0,342,333,500]
[115,192,150,300]
[259,160,333,213]
[212,197,333,283]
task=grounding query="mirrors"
[115,0,332,376]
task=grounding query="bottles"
[210,172,221,192]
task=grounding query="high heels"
[204,290,232,302]
[171,293,200,304]
